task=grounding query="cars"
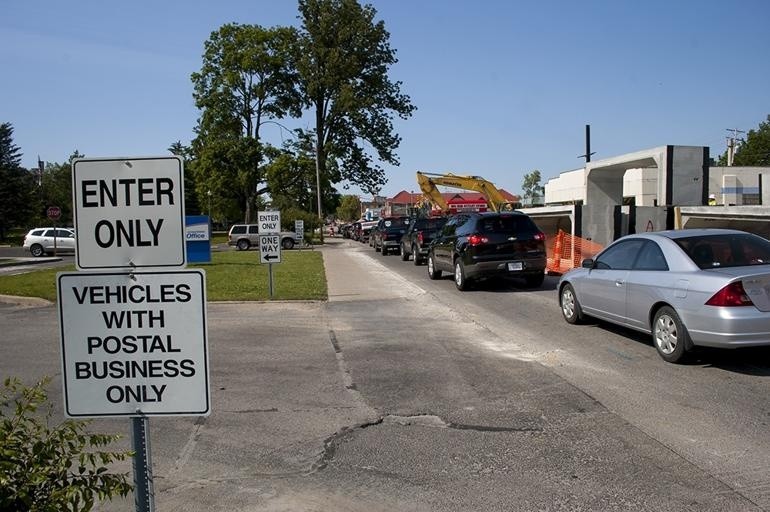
[557,228,770,365]
[23,228,76,257]
[342,216,412,255]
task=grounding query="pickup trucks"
[400,217,449,266]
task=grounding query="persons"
[329,225,334,237]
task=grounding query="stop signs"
[47,206,62,220]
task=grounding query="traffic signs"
[259,235,281,264]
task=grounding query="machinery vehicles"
[416,171,514,211]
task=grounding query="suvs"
[228,224,299,251]
[427,210,547,291]
